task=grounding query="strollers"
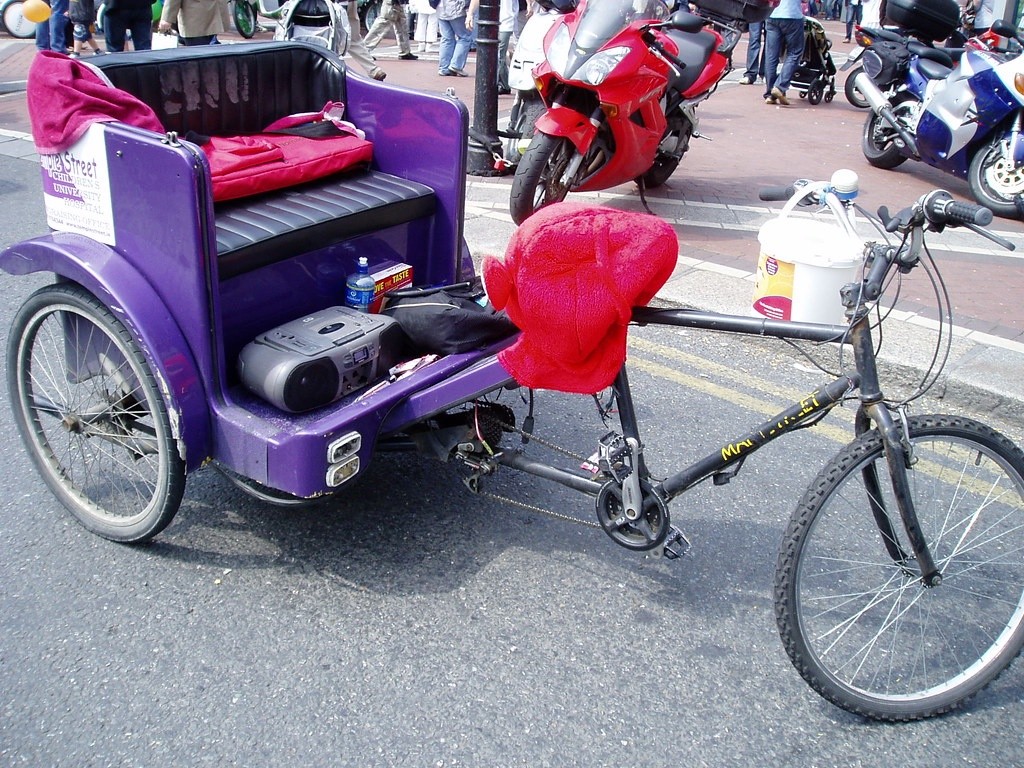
[778,14,836,105]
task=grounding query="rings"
[168,27,171,32]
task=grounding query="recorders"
[236,305,410,414]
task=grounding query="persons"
[738,0,863,104]
[338,0,547,96]
[34,0,230,58]
[963,0,1020,42]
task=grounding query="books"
[369,263,416,318]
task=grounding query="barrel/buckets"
[749,180,863,327]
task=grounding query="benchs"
[37,42,469,278]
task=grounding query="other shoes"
[399,53,417,60]
[439,65,468,77]
[498,86,510,95]
[374,72,386,81]
[739,76,750,84]
[842,38,850,43]
[766,87,790,105]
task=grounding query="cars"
[0,0,49,38]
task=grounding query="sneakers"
[93,50,106,56]
[69,53,80,59]
[418,42,440,53]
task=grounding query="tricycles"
[0,40,1024,725]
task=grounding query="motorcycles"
[839,24,1022,108]
[508,0,742,226]
[227,0,255,38]
[854,0,1024,217]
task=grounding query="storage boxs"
[688,0,783,24]
[885,0,963,45]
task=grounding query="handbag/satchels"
[382,274,522,354]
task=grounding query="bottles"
[345,257,376,312]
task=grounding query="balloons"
[22,0,53,24]
